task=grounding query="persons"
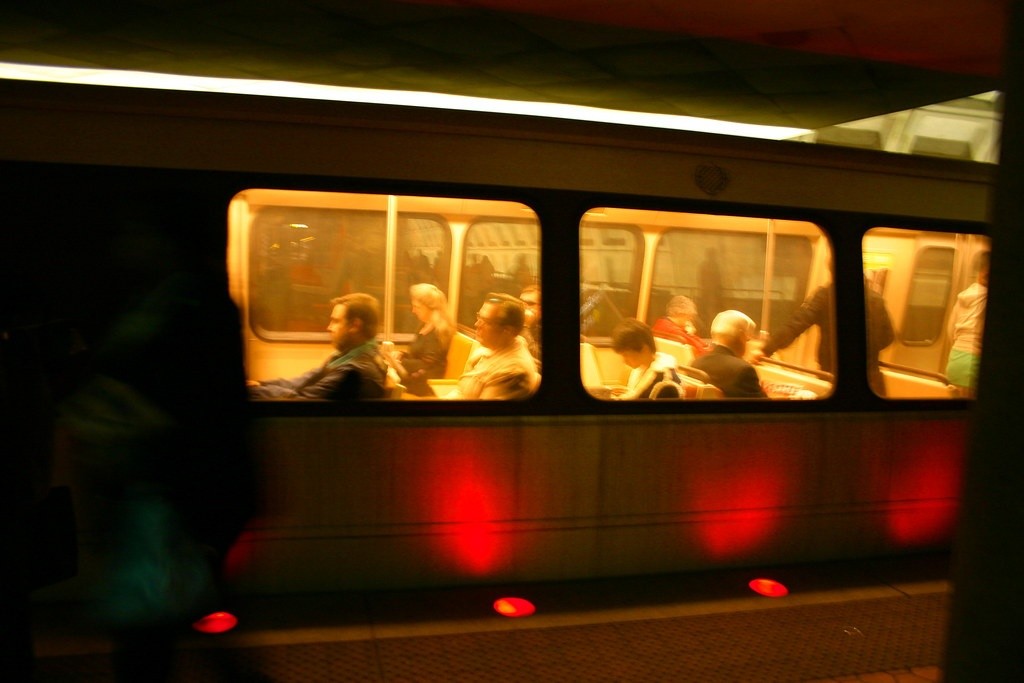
[611,318,681,400]
[749,265,895,395]
[393,282,452,397]
[245,291,387,404]
[453,291,538,403]
[518,286,542,361]
[652,295,709,359]
[688,309,770,399]
[945,247,989,400]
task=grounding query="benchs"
[876,360,960,398]
[381,321,542,397]
[585,330,835,400]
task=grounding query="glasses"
[474,312,504,327]
[520,299,543,307]
